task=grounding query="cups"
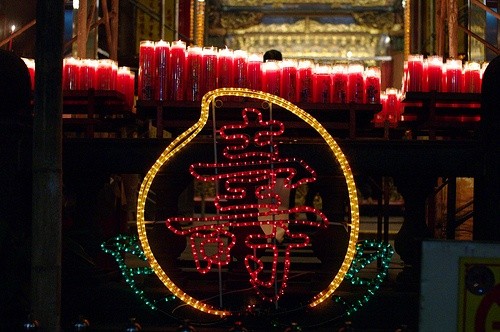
[401,53,489,121]
[138,40,381,107]
[22,56,135,113]
[369,87,403,128]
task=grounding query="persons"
[263,50,282,62]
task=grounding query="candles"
[15,38,490,121]
